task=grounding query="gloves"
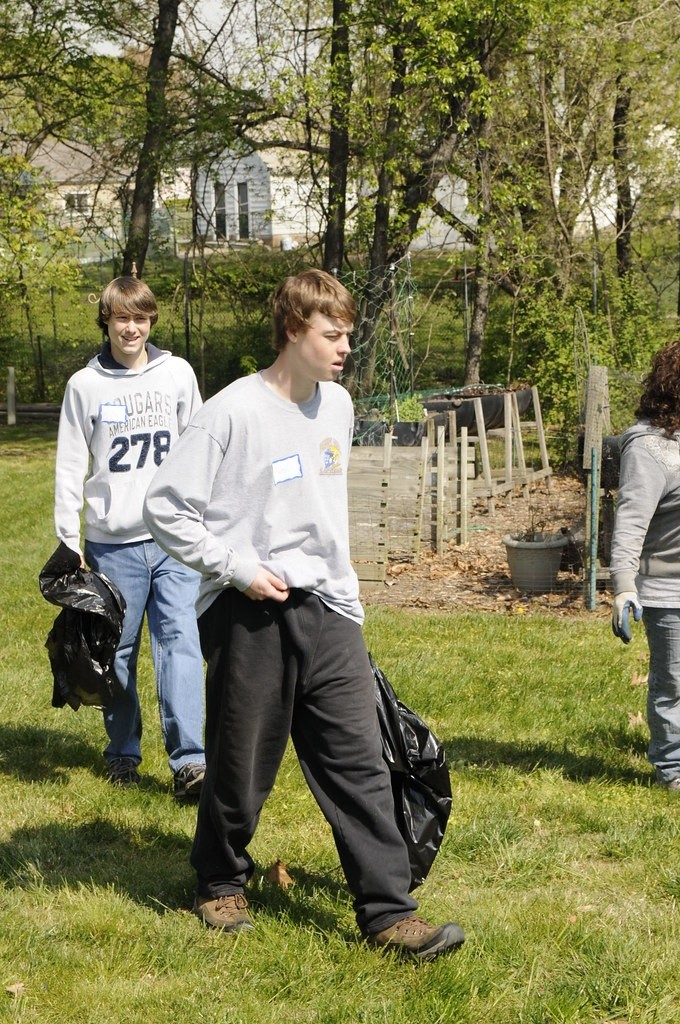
[611,591,643,645]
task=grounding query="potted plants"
[503,500,568,597]
[359,393,448,447]
[421,385,532,438]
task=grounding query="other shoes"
[668,778,680,789]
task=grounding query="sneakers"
[173,763,206,802]
[362,915,465,962]
[108,757,141,786]
[193,892,256,932]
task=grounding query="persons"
[55,276,204,800]
[142,269,465,960]
[611,341,680,789]
